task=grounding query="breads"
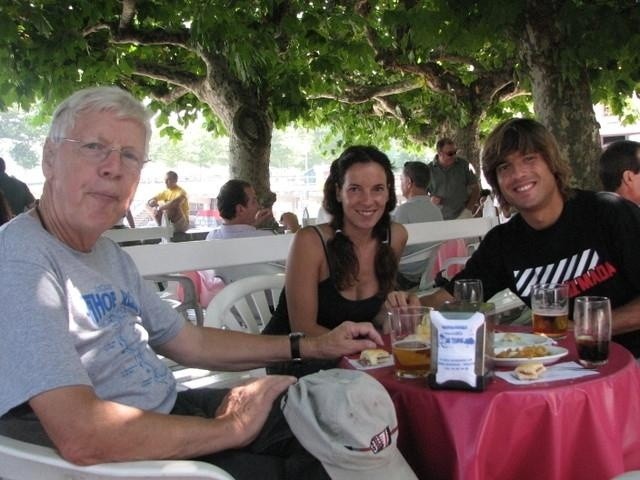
[514,362,547,379]
[358,348,390,367]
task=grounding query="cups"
[529,284,570,341]
[302,218,318,227]
[453,278,485,308]
[284,229,292,235]
[574,296,612,367]
[388,305,433,381]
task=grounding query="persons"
[472,188,500,218]
[0,189,14,227]
[424,136,482,222]
[203,179,302,242]
[0,156,40,219]
[390,160,446,293]
[0,82,386,480]
[416,113,640,367]
[597,140,640,211]
[111,208,136,230]
[258,143,423,381]
[146,170,191,233]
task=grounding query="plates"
[493,347,570,367]
[492,331,553,349]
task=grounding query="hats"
[280,367,418,480]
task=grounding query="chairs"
[144,242,489,394]
[0,433,236,480]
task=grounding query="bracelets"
[288,330,307,362]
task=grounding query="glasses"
[60,136,150,171]
[440,151,457,156]
